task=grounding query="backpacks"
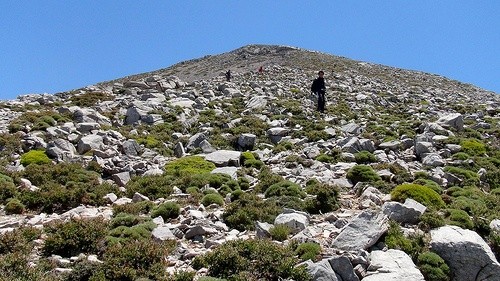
[311,78,321,92]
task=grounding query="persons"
[225,70,231,82]
[314,71,326,116]
